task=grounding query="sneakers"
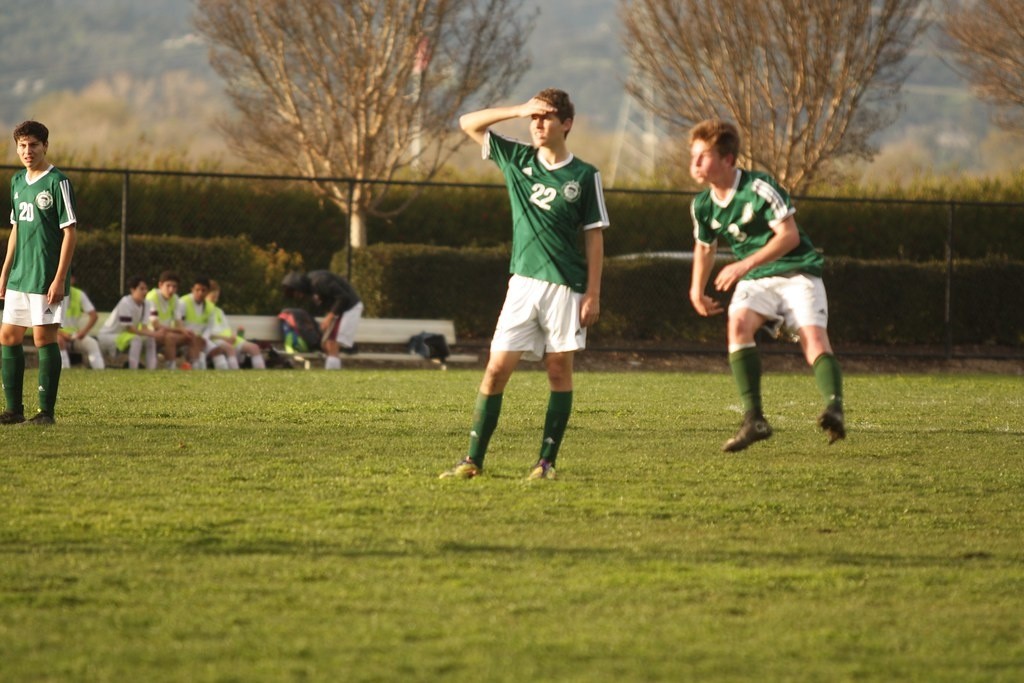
[723,414,774,453]
[0,409,25,424]
[21,410,56,426]
[818,407,847,440]
[440,456,481,480]
[530,460,556,481]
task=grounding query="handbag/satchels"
[409,331,450,359]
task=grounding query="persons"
[0,121,78,425]
[52,262,105,369]
[146,271,266,369]
[440,89,609,481]
[98,277,164,371]
[688,118,846,453]
[282,269,365,371]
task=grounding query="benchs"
[0,312,479,369]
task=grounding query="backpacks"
[280,306,323,356]
